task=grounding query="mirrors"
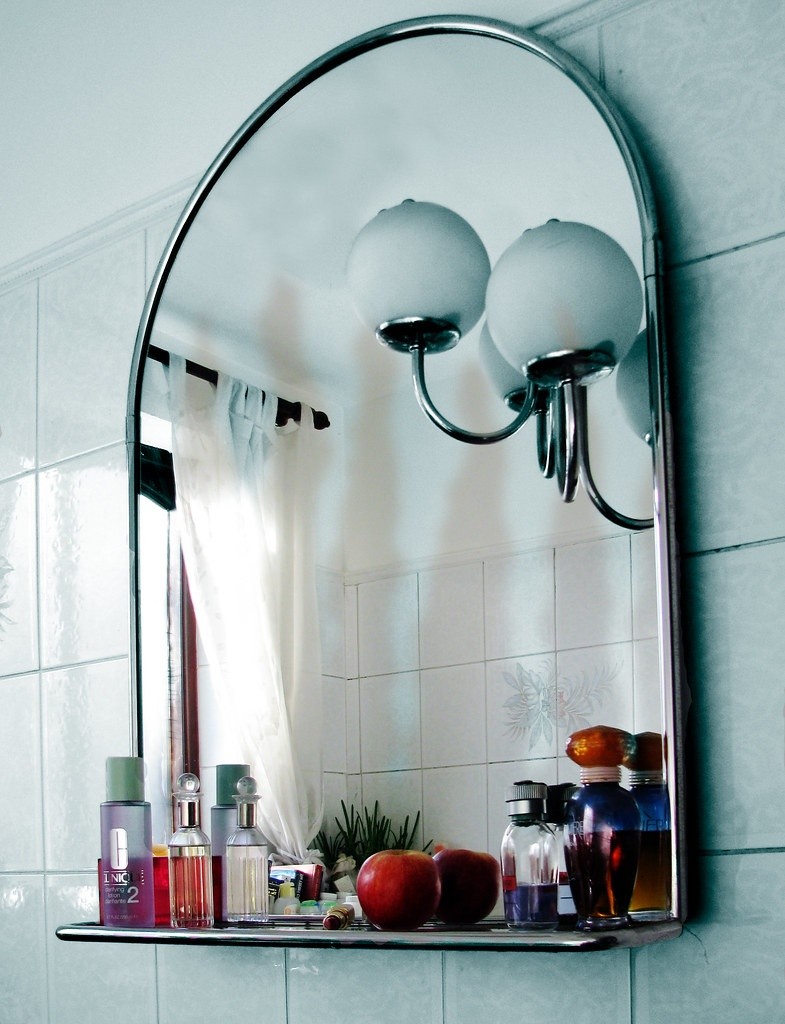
[125,12,688,923]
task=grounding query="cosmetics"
[556,728,643,933]
[167,773,216,930]
[223,777,269,923]
[624,731,672,922]
[100,755,153,929]
[499,779,559,932]
[321,903,355,930]
[211,764,251,926]
[272,882,365,919]
[540,782,580,927]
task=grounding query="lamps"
[342,197,655,530]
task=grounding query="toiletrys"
[270,862,360,920]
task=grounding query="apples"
[355,849,441,930]
[431,849,500,926]
